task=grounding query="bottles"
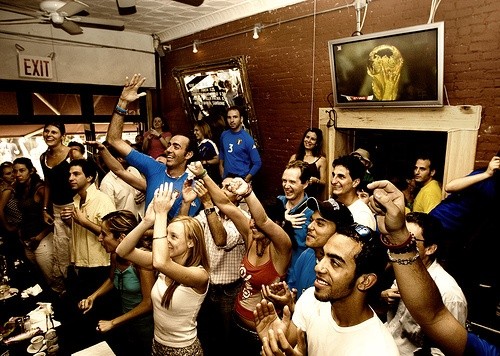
[45,313,61,355]
[0,256,13,286]
[0,335,11,356]
[23,315,32,333]
[15,318,24,333]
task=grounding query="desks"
[0,252,117,356]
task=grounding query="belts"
[237,324,260,341]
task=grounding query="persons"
[0,74,500,356]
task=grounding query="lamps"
[192,40,200,54]
[14,42,57,78]
[253,24,262,39]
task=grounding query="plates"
[0,288,19,300]
[27,340,47,353]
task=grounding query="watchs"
[204,207,216,214]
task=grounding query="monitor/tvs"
[326,21,444,109]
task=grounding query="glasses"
[349,221,376,250]
[354,155,364,160]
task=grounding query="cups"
[33,352,46,356]
[0,285,10,297]
[30,336,44,349]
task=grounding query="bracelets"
[387,247,420,265]
[113,108,129,117]
[242,183,252,197]
[43,206,47,212]
[379,232,412,247]
[114,105,129,113]
[198,169,207,178]
[112,319,115,326]
[152,235,167,240]
[388,234,418,254]
[156,134,163,139]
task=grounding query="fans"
[0,0,124,35]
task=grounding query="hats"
[349,148,373,169]
[307,196,355,235]
